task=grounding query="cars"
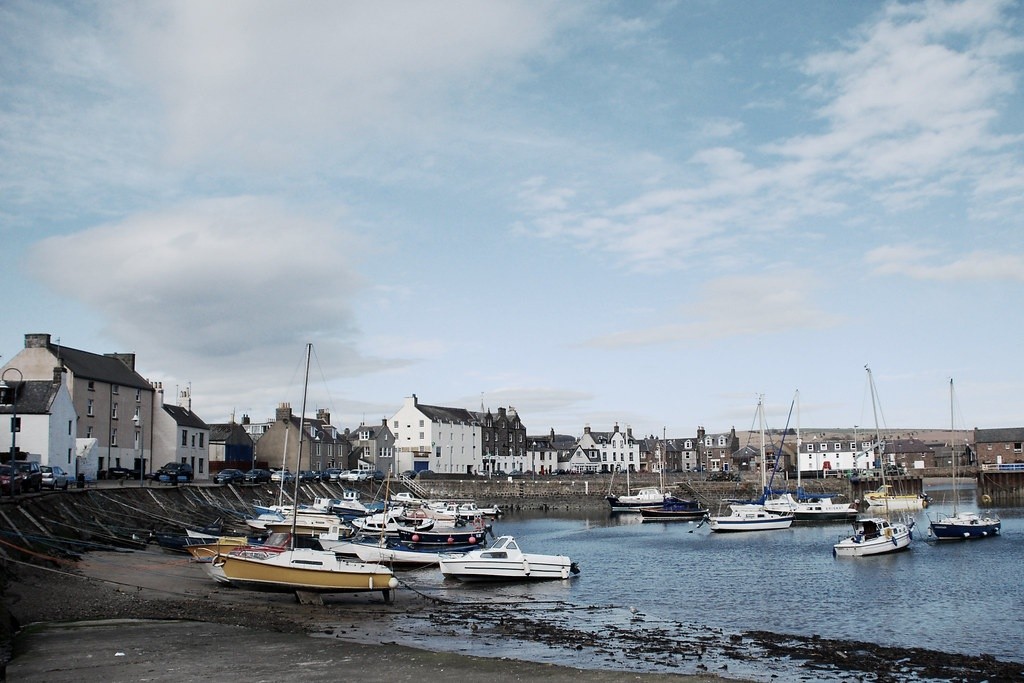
[6,460,43,493]
[477,467,701,477]
[40,465,69,491]
[153,462,195,483]
[214,469,434,484]
[0,465,24,496]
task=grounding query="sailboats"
[926,379,1001,541]
[604,424,710,518]
[350,468,464,570]
[696,392,797,534]
[833,363,917,557]
[763,388,859,523]
[212,343,398,606]
[251,427,293,515]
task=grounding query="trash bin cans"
[133,469,141,480]
[97,470,107,480]
[77,473,85,488]
[315,475,321,482]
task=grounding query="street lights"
[131,404,149,487]
[245,423,257,484]
[532,437,536,481]
[0,368,23,499]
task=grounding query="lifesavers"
[473,518,485,530]
[471,503,477,511]
[913,500,917,503]
[840,497,847,503]
[885,528,892,539]
[871,496,875,500]
[849,503,857,510]
[982,495,991,504]
[970,519,978,524]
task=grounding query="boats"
[863,484,933,509]
[154,491,580,582]
